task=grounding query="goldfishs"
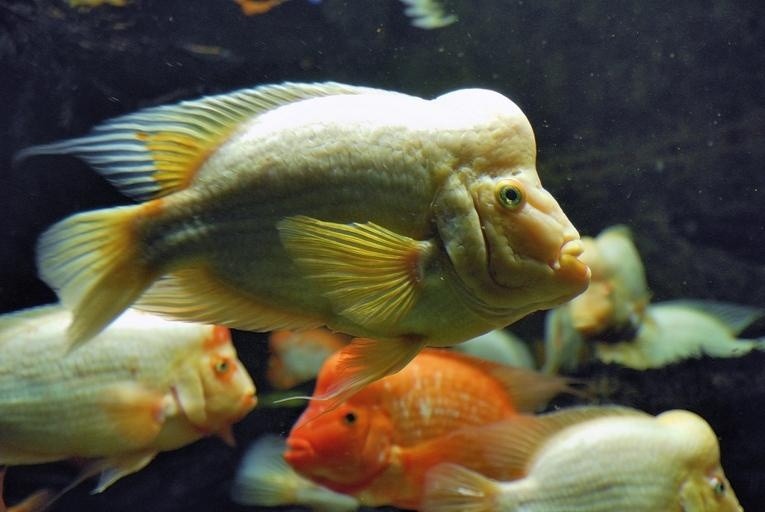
[0,302,260,512]
[13,79,594,434]
[228,222,765,512]
